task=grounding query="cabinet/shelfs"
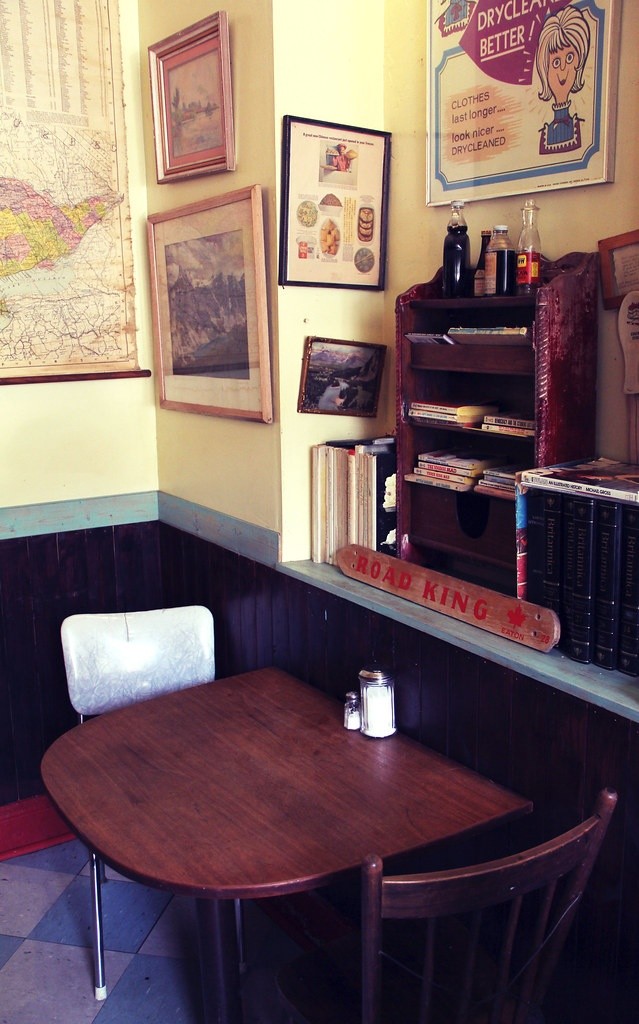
[395,252,601,575]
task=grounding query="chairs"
[360,784,618,1024]
[59,605,248,1001]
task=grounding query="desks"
[40,666,535,1024]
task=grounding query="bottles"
[485,224,516,296]
[443,201,472,298]
[343,691,361,731]
[474,231,491,297]
[359,664,396,738]
[515,198,542,295]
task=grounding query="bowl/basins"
[319,204,343,216]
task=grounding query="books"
[404,328,532,345]
[404,444,639,677]
[310,435,396,567]
[407,400,537,438]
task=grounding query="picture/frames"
[597,229,639,310]
[143,182,277,426]
[147,10,237,185]
[423,0,623,209]
[277,115,395,293]
[297,336,389,419]
